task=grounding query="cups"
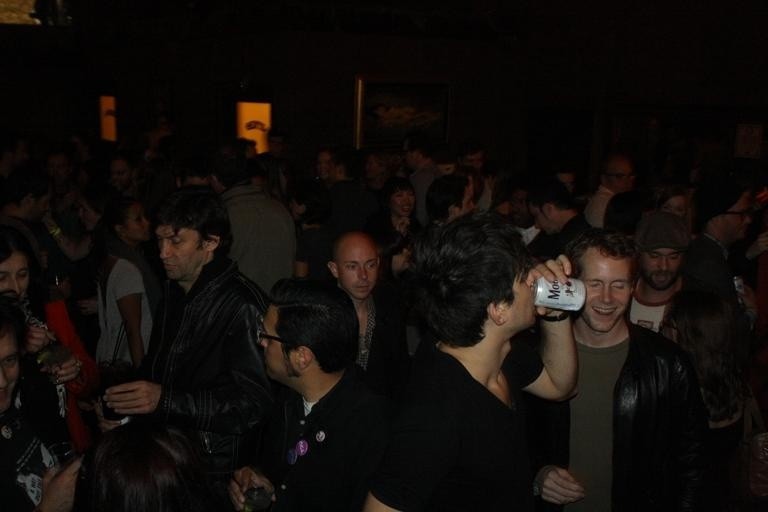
[42,348,71,385]
[96,358,133,422]
[242,480,276,512]
[48,441,77,475]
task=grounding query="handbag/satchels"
[727,371,768,498]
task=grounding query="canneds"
[532,277,586,311]
[733,275,745,303]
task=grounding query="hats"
[635,214,691,254]
[694,174,746,224]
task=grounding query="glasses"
[254,313,285,345]
[604,170,637,179]
[718,206,753,217]
